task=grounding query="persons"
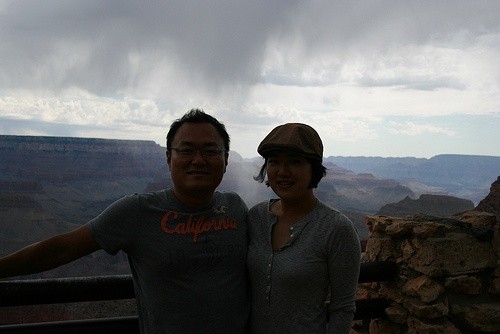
[0,111,249,334]
[247,124,361,334]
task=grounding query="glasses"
[170,147,227,158]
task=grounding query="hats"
[257,123,323,164]
